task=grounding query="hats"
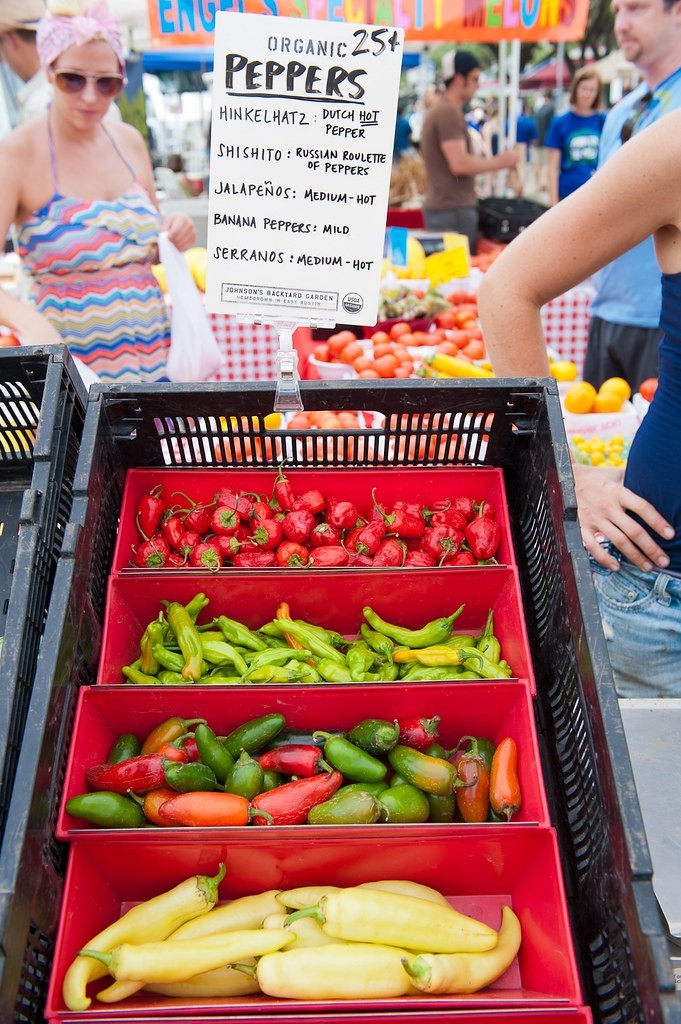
[36,0,123,73]
[0,0,45,33]
[441,49,485,82]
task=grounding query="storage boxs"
[0,341,681,1024]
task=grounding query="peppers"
[133,456,500,567]
[63,863,522,1009]
[121,592,513,684]
[66,714,522,826]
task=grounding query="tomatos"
[213,288,660,467]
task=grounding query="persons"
[504,90,555,190]
[393,49,518,256]
[582,0,681,403]
[0,0,196,431]
[476,106,681,699]
[167,154,195,198]
[542,70,607,208]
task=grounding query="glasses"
[48,65,129,98]
[14,28,35,40]
